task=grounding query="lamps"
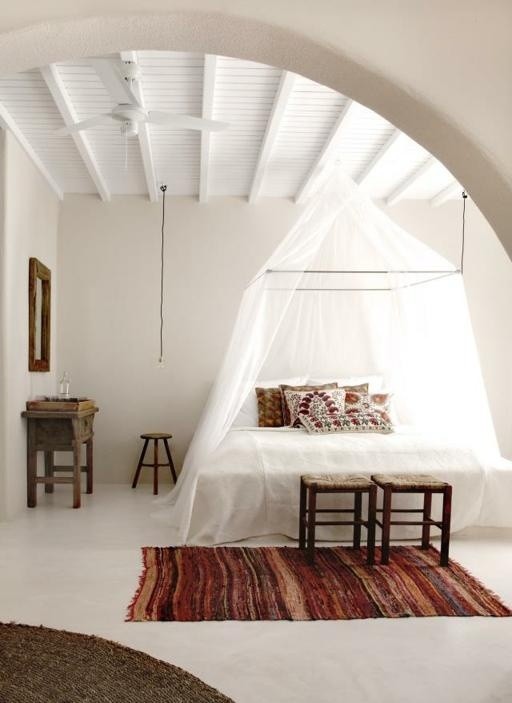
[111,118,143,137]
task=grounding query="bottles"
[58,372,71,393]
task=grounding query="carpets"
[124,542,510,621]
[0,617,238,702]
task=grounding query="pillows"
[255,381,397,435]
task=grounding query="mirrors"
[28,255,54,374]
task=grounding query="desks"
[22,396,98,510]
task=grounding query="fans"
[53,50,228,140]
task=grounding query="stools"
[299,471,377,565]
[367,471,453,566]
[132,431,179,494]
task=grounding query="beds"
[153,159,512,545]
